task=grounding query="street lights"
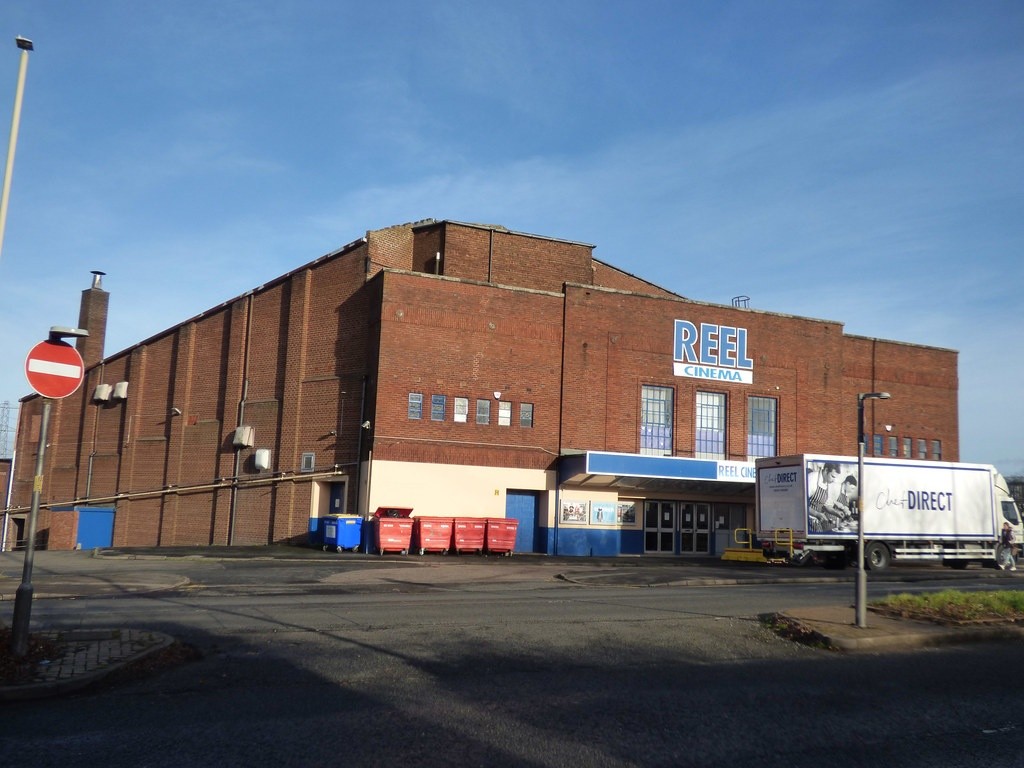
[853,387,892,630]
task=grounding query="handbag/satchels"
[1011,544,1021,556]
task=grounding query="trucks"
[754,451,1024,573]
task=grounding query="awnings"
[560,451,757,489]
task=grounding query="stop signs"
[24,337,85,400]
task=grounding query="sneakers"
[1009,567,1016,572]
[998,563,1005,570]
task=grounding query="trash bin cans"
[451,516,487,554]
[373,505,414,555]
[484,517,520,556]
[320,512,363,553]
[412,516,453,555]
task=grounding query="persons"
[808,462,857,533]
